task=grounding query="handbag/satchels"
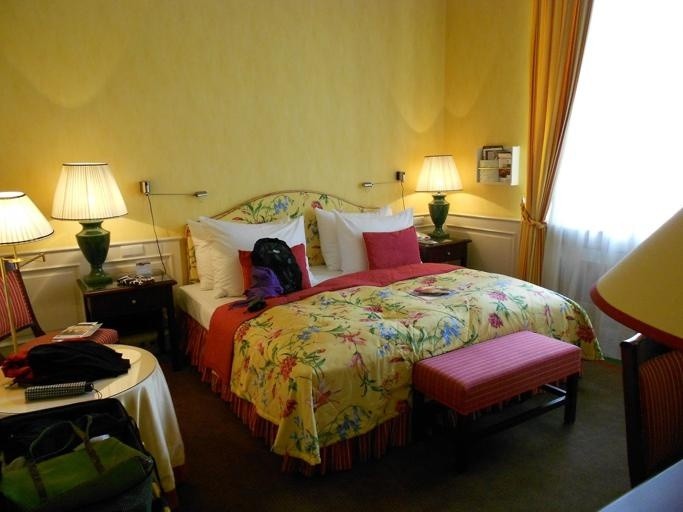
[0,398,169,512]
[27,340,130,385]
[245,266,284,299]
[0,437,154,512]
[252,238,302,294]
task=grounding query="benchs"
[411,329,580,473]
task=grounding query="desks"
[3,342,178,497]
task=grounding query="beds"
[178,190,582,480]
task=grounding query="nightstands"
[75,265,178,368]
[417,233,471,267]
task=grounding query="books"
[478,144,512,185]
[51,321,103,342]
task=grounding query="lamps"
[0,161,128,285]
[415,155,464,239]
[589,207,683,353]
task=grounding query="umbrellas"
[26,381,103,402]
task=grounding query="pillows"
[183,206,424,299]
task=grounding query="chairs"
[619,330,682,487]
[0,255,120,353]
